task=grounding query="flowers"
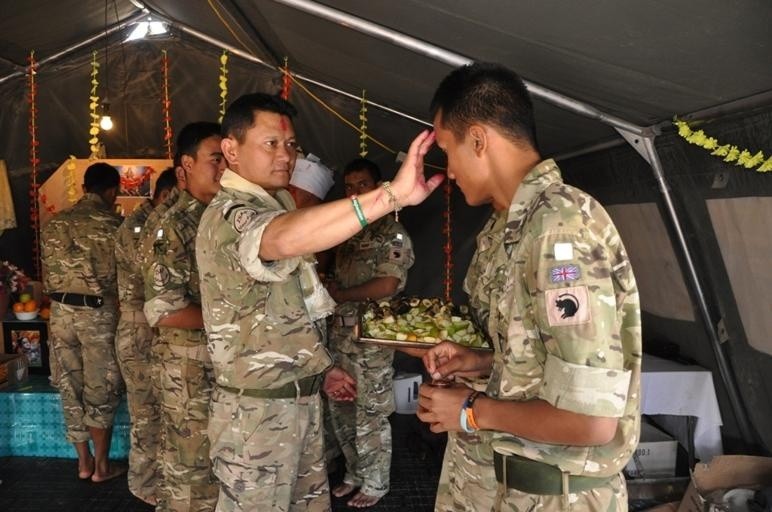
[0,260,32,314]
[671,118,772,172]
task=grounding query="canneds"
[427,381,450,424]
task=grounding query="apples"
[19,294,30,302]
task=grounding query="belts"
[167,341,209,364]
[218,376,324,400]
[493,451,619,495]
[49,292,118,307]
[333,315,356,327]
[121,309,146,324]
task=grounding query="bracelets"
[383,181,403,222]
[351,192,369,230]
[459,390,487,434]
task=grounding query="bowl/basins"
[15,308,40,320]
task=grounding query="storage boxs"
[677,455,772,512]
[625,420,678,478]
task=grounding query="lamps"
[100,0,129,157]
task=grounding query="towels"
[220,168,337,321]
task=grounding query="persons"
[324,159,415,508]
[288,145,336,284]
[112,168,177,505]
[41,162,127,482]
[413,61,644,512]
[137,118,228,512]
[194,93,445,512]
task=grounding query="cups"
[427,381,452,389]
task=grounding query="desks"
[623,352,725,501]
[2,315,50,375]
